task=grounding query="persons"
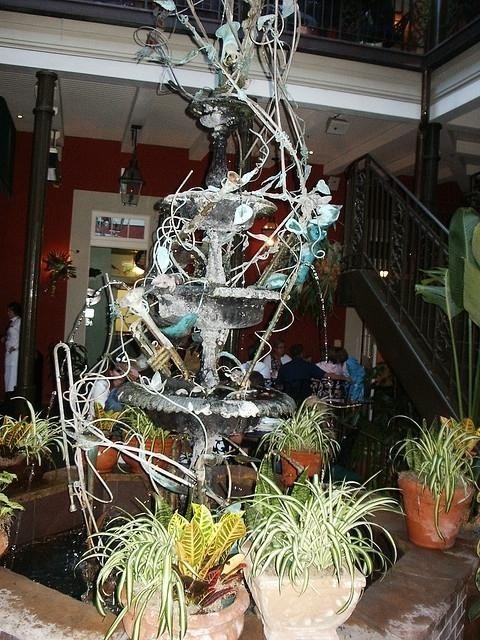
[1,303,23,410]
[79,340,364,473]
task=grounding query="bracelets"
[11,347,15,351]
[4,336,8,341]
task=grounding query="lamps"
[118,123,145,207]
